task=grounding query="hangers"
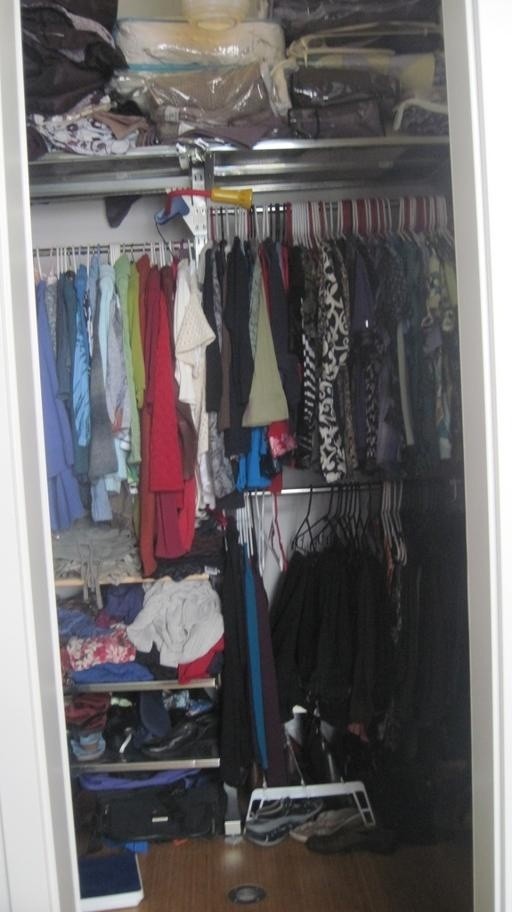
[206,191,455,255]
[230,477,412,576]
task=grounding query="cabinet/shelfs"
[48,571,225,774]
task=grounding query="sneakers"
[246,798,328,846]
[306,823,378,852]
[290,808,356,842]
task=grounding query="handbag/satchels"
[102,777,228,840]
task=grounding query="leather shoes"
[142,713,219,756]
